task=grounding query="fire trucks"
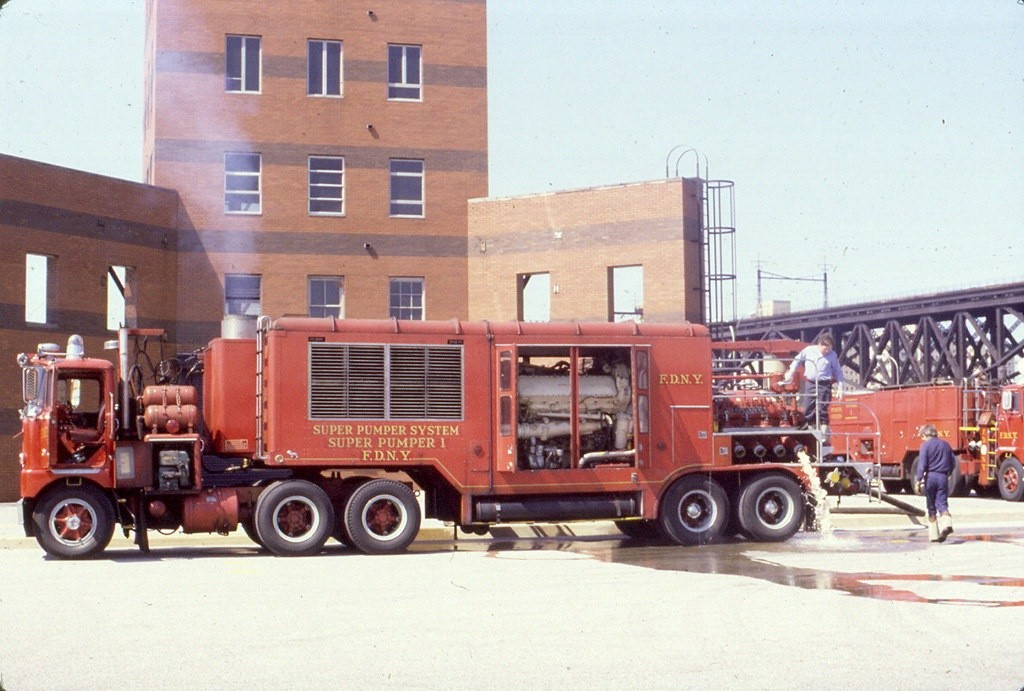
[13,317,882,553]
[824,376,1024,503]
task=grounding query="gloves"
[915,483,922,495]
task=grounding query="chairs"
[67,405,105,442]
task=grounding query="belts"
[807,381,829,385]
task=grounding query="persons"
[784,334,841,444]
[916,425,955,543]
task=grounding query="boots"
[929,519,938,541]
[937,512,953,542]
